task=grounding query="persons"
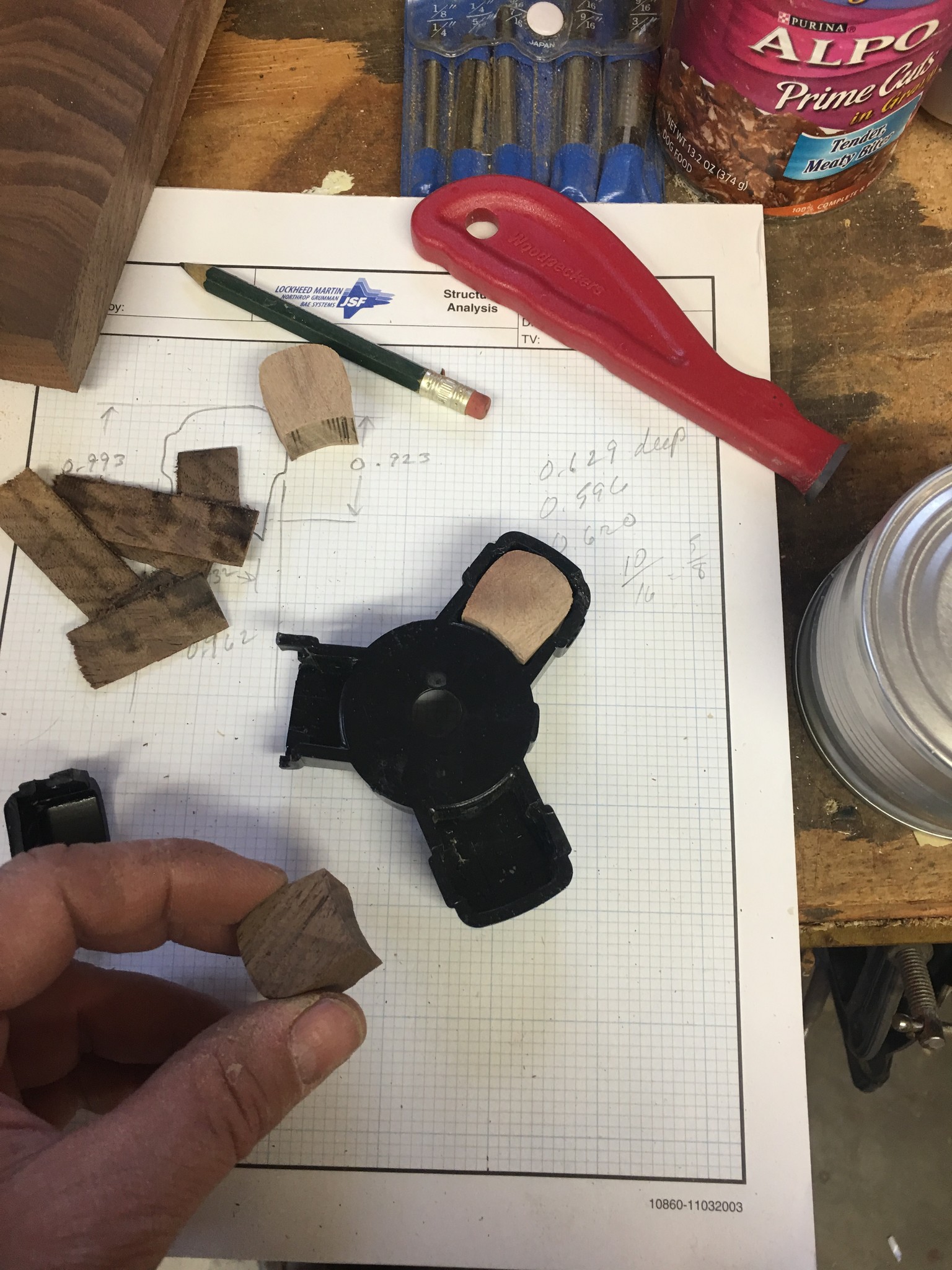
[0,836,367,1269]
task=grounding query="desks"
[155,0,952,948]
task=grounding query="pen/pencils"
[177,261,493,417]
